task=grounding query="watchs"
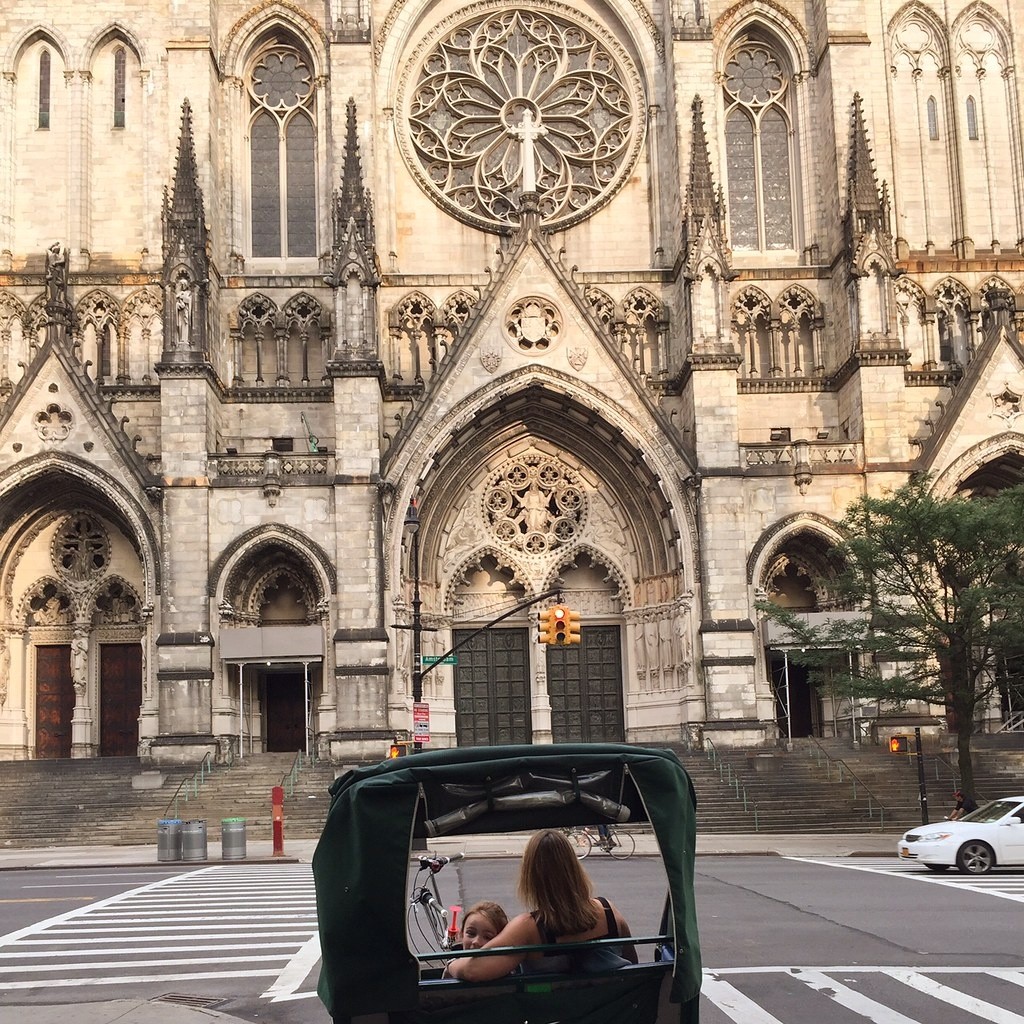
[446,958,456,969]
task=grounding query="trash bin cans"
[157,821,182,861]
[183,819,208,861]
[221,817,247,860]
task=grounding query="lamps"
[317,446,329,452]
[817,430,828,439]
[770,433,782,440]
[226,447,237,454]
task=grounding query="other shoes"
[606,839,616,852]
[594,836,606,845]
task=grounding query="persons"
[71,631,88,686]
[460,900,508,949]
[441,829,639,983]
[592,825,617,853]
[46,241,64,285]
[948,790,978,821]
[635,607,688,670]
